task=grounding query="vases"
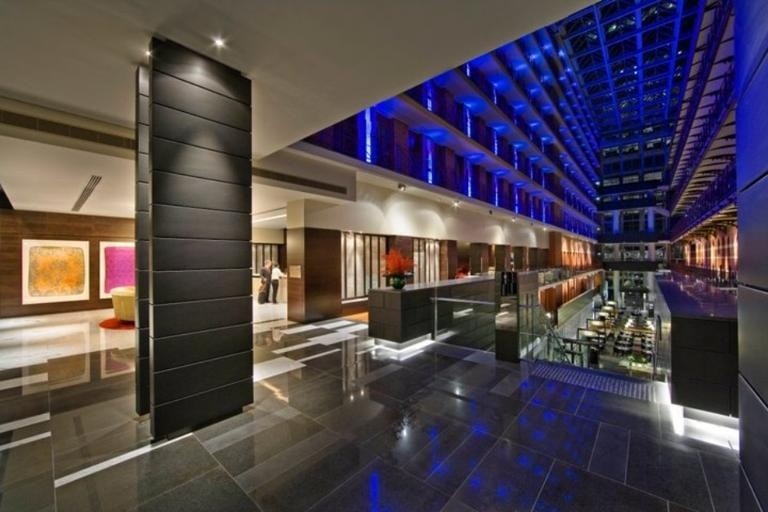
[382,274,414,291]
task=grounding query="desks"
[612,328,653,362]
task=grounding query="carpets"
[98,318,137,330]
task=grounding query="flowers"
[381,248,417,274]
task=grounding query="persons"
[259,259,274,305]
[270,262,287,304]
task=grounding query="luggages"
[259,284,267,304]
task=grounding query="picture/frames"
[21,238,90,306]
[98,240,136,299]
